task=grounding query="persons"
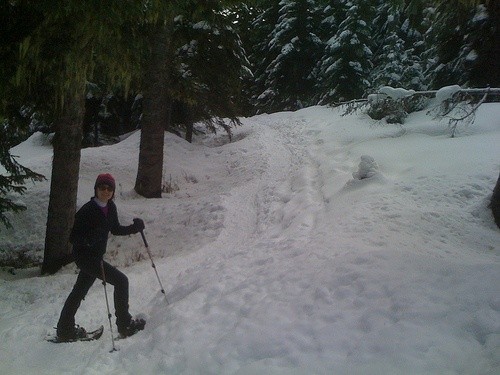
[56,174,145,339]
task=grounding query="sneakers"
[118,319,145,337]
[57,327,86,339]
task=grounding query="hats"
[94,174,115,192]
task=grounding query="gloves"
[86,238,107,251]
[130,218,145,234]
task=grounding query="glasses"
[97,184,113,191]
